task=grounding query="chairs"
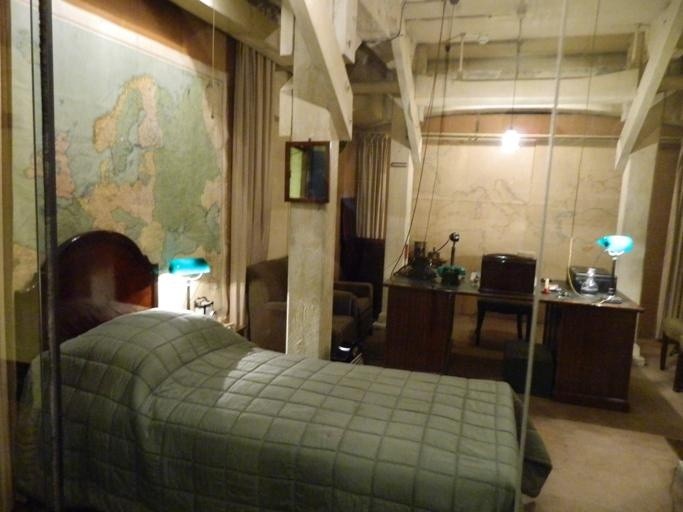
[658,313,683,392]
[472,250,537,345]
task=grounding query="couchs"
[233,249,374,365]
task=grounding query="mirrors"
[282,140,331,205]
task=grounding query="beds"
[17,227,557,511]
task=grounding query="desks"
[381,241,646,417]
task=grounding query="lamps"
[500,12,530,155]
[593,234,638,304]
[165,255,215,317]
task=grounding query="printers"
[567,266,616,295]
